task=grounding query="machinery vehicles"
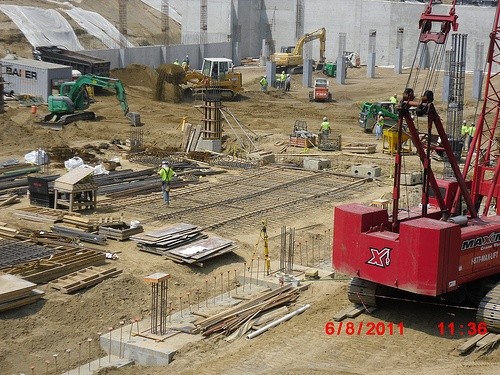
[269,26,327,76]
[331,0,500,335]
[178,57,245,102]
[309,78,332,103]
[322,50,362,77]
[32,72,141,131]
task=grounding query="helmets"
[378,111,383,115]
[287,74,290,77]
[162,160,169,164]
[282,71,285,73]
[323,117,328,120]
[264,75,267,78]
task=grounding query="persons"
[159,161,176,208]
[462,120,477,153]
[175,55,190,72]
[376,111,385,140]
[260,71,291,92]
[389,94,399,115]
[319,116,332,139]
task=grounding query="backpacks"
[376,125,383,137]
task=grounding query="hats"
[463,120,466,124]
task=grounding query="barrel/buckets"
[130,220,140,229]
[31,106,36,114]
